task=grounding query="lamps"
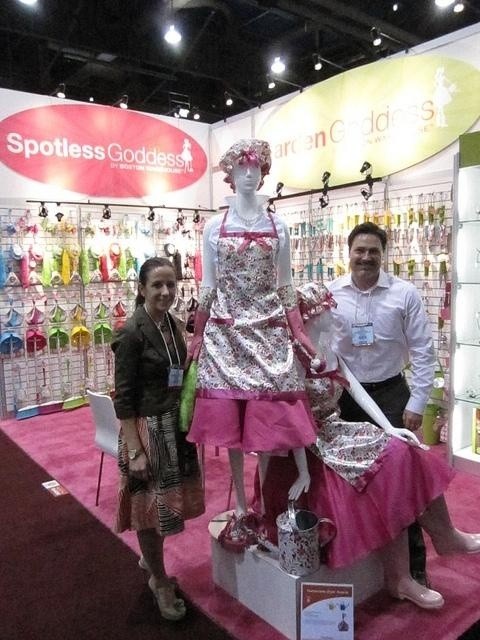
[28,197,217,228]
[173,106,200,120]
[270,47,285,74]
[314,54,323,70]
[265,161,383,215]
[57,83,66,99]
[120,95,129,109]
[265,74,276,89]
[89,97,94,102]
[224,92,233,107]
[164,1,182,44]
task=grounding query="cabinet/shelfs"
[448,130,480,480]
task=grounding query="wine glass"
[473,202,480,403]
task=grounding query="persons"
[252,279,480,610]
[109,257,206,622]
[184,136,320,520]
[303,220,434,589]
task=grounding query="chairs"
[87,389,122,507]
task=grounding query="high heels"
[137,556,188,621]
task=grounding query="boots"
[379,528,445,610]
[421,493,480,555]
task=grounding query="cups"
[328,599,349,611]
[423,405,443,446]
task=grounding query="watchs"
[127,447,144,461]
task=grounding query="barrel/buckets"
[277,498,335,578]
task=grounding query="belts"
[359,373,404,391]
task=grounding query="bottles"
[337,613,349,632]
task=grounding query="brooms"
[1,320,118,421]
